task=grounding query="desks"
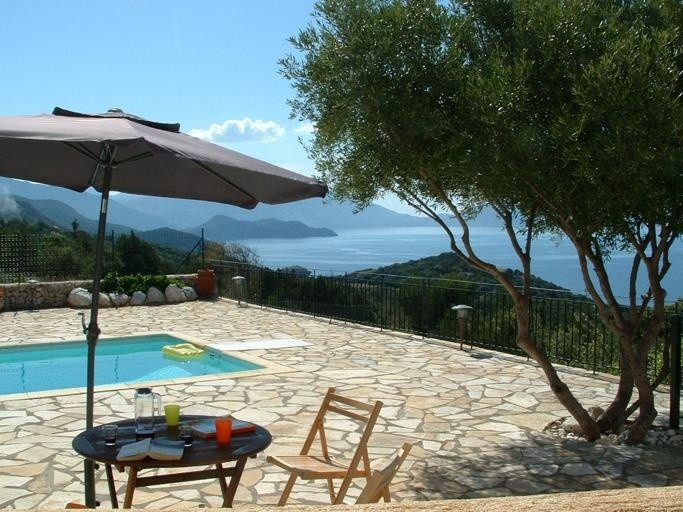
[71,414,272,508]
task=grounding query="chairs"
[354,442,414,506]
[266,386,384,505]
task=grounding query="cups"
[102,425,118,446]
[215,420,232,444]
[134,388,162,433]
[179,426,193,446]
[164,405,179,426]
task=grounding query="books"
[115,437,186,461]
[188,414,258,439]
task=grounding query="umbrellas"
[0,102,330,507]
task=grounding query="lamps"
[232,276,246,305]
[452,303,474,351]
